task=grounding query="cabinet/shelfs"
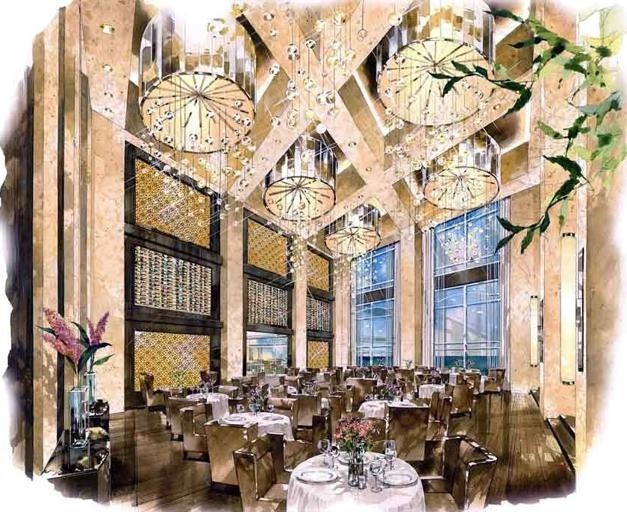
[41,427,111,507]
[71,397,110,435]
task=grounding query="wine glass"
[189,379,217,400]
[384,440,397,471]
[370,460,382,492]
[317,437,339,469]
[236,404,274,419]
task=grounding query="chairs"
[133,364,514,511]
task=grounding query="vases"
[83,372,96,403]
[66,387,85,448]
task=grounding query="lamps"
[134,1,539,314]
[529,294,543,367]
[557,231,579,388]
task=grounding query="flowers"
[68,309,116,402]
[32,303,111,391]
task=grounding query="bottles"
[354,448,368,488]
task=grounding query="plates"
[296,467,336,482]
[380,469,416,485]
[339,452,372,464]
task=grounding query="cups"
[374,455,386,473]
